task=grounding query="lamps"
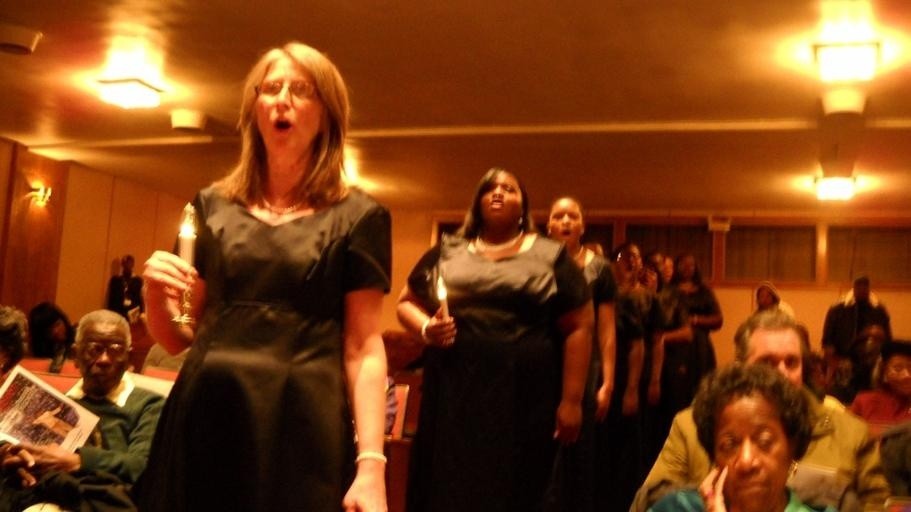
[814,172,855,204]
[166,106,207,131]
[23,179,54,210]
[811,13,884,84]
[0,19,47,54]
[85,31,169,111]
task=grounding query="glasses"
[254,82,317,101]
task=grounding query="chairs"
[17,351,183,400]
[378,327,424,510]
